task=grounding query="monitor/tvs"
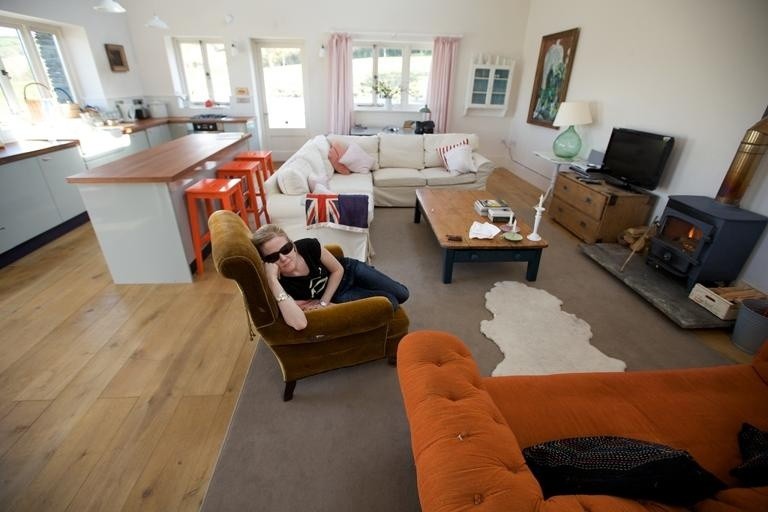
[600,126,675,194]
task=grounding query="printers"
[570,149,605,176]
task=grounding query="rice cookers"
[146,98,169,120]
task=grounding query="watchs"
[320,301,326,307]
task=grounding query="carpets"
[200,207,749,510]
[478,276,628,378]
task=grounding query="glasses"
[259,242,294,264]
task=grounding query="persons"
[251,224,409,331]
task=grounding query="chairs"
[208,210,408,401]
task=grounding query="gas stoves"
[187,114,229,131]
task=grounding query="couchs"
[396,330,768,512]
[261,130,497,254]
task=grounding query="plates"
[503,231,524,241]
[500,223,521,232]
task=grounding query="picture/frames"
[103,44,129,72]
[527,28,580,129]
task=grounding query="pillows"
[520,437,732,505]
[339,143,376,174]
[734,423,767,487]
[313,184,332,194]
[445,145,477,176]
[327,141,350,175]
[308,173,331,191]
[436,138,467,172]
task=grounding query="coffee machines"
[114,98,147,121]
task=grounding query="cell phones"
[448,234,462,241]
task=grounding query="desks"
[66,130,251,285]
[350,128,413,135]
[531,152,590,211]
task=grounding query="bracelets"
[276,292,291,302]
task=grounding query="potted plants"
[361,78,423,110]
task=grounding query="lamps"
[552,102,592,158]
[318,40,326,59]
[230,44,238,56]
[144,0,171,29]
[92,1,126,14]
[421,104,432,121]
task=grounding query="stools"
[185,150,275,273]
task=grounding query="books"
[474,199,516,224]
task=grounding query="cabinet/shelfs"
[223,120,259,151]
[549,172,652,245]
[38,142,91,222]
[464,56,516,121]
[168,122,194,138]
[0,155,63,253]
[146,122,171,147]
